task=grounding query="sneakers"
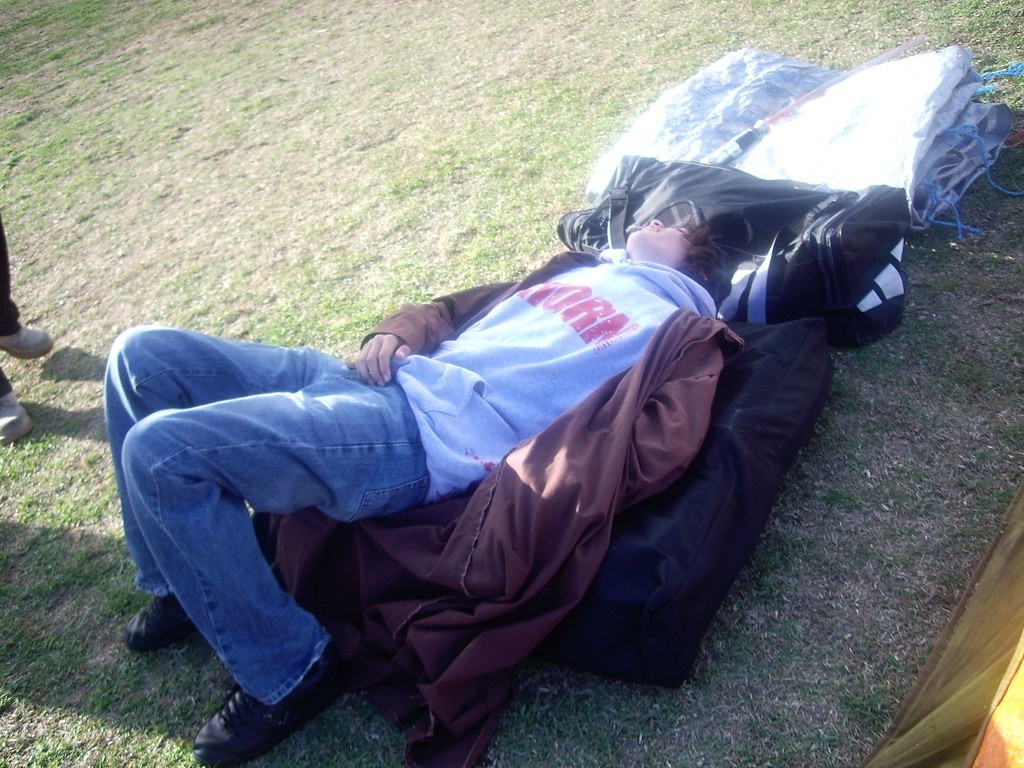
[0,391,33,445]
[0,327,54,359]
[124,592,201,651]
[193,656,339,768]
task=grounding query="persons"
[0,213,55,442]
[103,217,745,768]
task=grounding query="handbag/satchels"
[568,315,834,685]
[557,154,911,349]
